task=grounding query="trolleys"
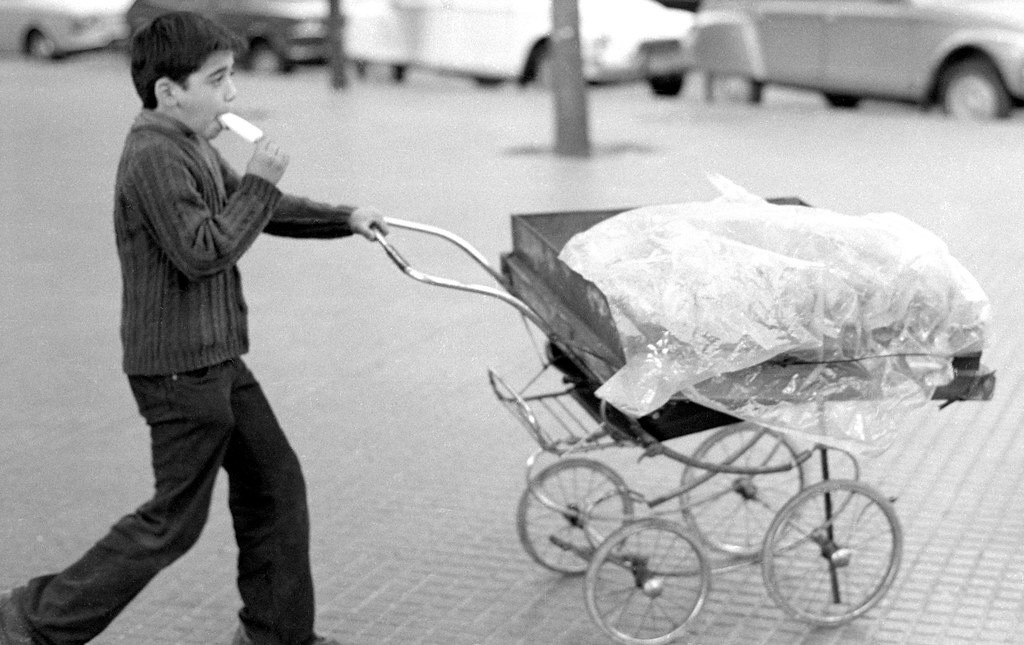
[372,194,999,645]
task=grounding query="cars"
[0,1,1024,123]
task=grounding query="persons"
[0,9,390,645]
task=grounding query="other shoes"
[0,590,36,645]
[233,623,340,645]
